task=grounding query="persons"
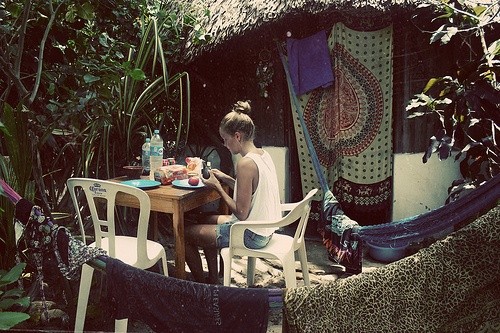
[184,102,282,286]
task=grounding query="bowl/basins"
[366,241,408,260]
[123,166,144,179]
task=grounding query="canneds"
[163,158,175,166]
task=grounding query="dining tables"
[94,175,229,280]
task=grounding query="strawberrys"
[161,166,188,185]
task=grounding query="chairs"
[67,178,168,333]
[220,188,318,290]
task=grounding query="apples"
[188,176,199,186]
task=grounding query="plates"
[119,179,161,190]
[172,179,205,188]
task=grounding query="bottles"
[142,138,151,174]
[207,162,212,169]
[150,130,163,180]
